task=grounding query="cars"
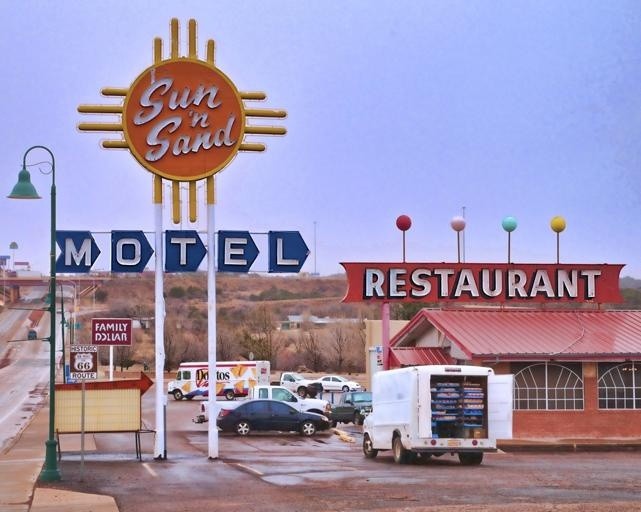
[317,375,361,392]
[216,400,330,436]
[28,331,37,340]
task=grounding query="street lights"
[5,146,63,483]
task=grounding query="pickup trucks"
[331,391,373,427]
[191,385,332,432]
[271,372,324,398]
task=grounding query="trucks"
[362,364,515,466]
[167,360,270,401]
